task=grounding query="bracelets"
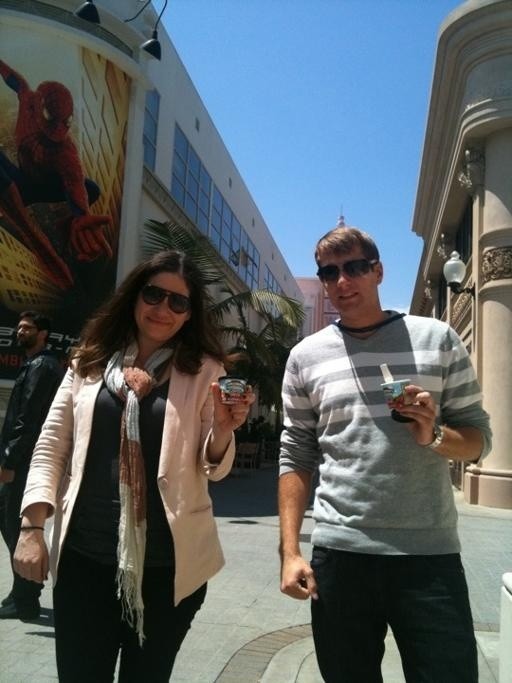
[16,525,46,532]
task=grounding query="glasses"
[316,257,378,285]
[140,282,194,314]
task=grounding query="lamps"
[120,0,170,62]
[73,0,104,25]
[442,248,475,303]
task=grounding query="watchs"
[421,422,443,450]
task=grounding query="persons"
[1,56,115,269]
[0,310,66,622]
[276,224,495,681]
[12,248,258,683]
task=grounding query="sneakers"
[0,592,41,621]
